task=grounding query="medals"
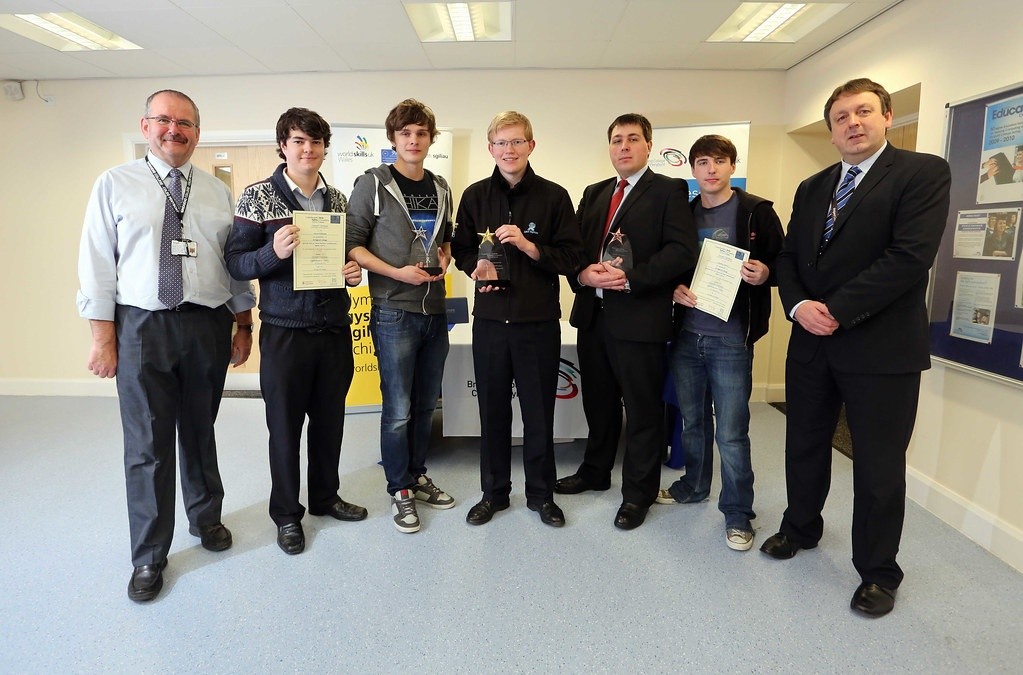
[602,227,633,269]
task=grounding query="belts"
[177,303,203,311]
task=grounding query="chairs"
[444,297,470,324]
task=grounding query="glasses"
[147,114,198,130]
[493,138,528,148]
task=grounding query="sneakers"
[656,488,677,504]
[725,528,754,550]
[391,488,421,534]
[412,473,456,509]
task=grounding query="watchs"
[238,324,253,331]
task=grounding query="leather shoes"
[277,521,305,555]
[526,498,565,526]
[128,558,168,601]
[309,498,368,522]
[188,519,232,552]
[615,500,648,529]
[851,582,896,619]
[466,496,510,525]
[554,474,612,494]
[758,529,819,559]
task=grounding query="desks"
[377,321,686,470]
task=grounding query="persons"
[451,111,574,528]
[555,113,689,529]
[1012,145,1023,182]
[760,78,951,618]
[980,157,999,183]
[76,90,255,599]
[984,212,1016,256]
[344,97,455,532]
[223,107,367,556]
[656,134,786,552]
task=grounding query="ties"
[597,179,629,263]
[158,169,183,309]
[819,165,862,265]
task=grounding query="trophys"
[409,225,442,275]
[476,227,511,288]
